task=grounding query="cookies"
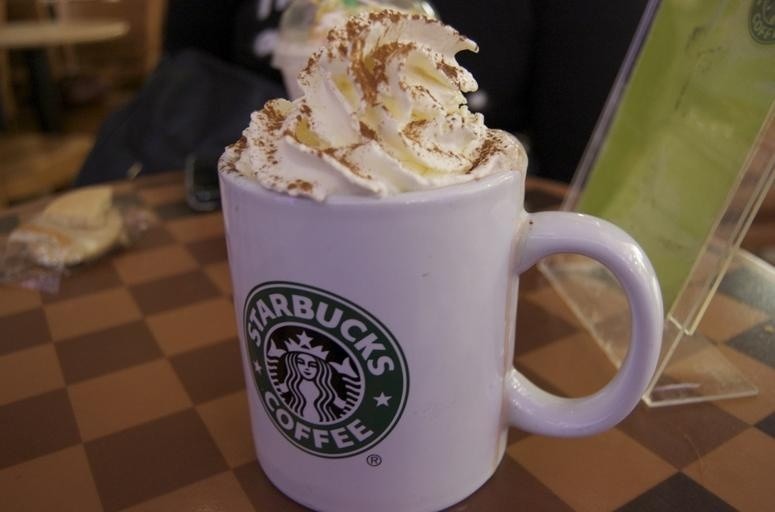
[11,184,124,268]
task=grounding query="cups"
[218,131,664,512]
